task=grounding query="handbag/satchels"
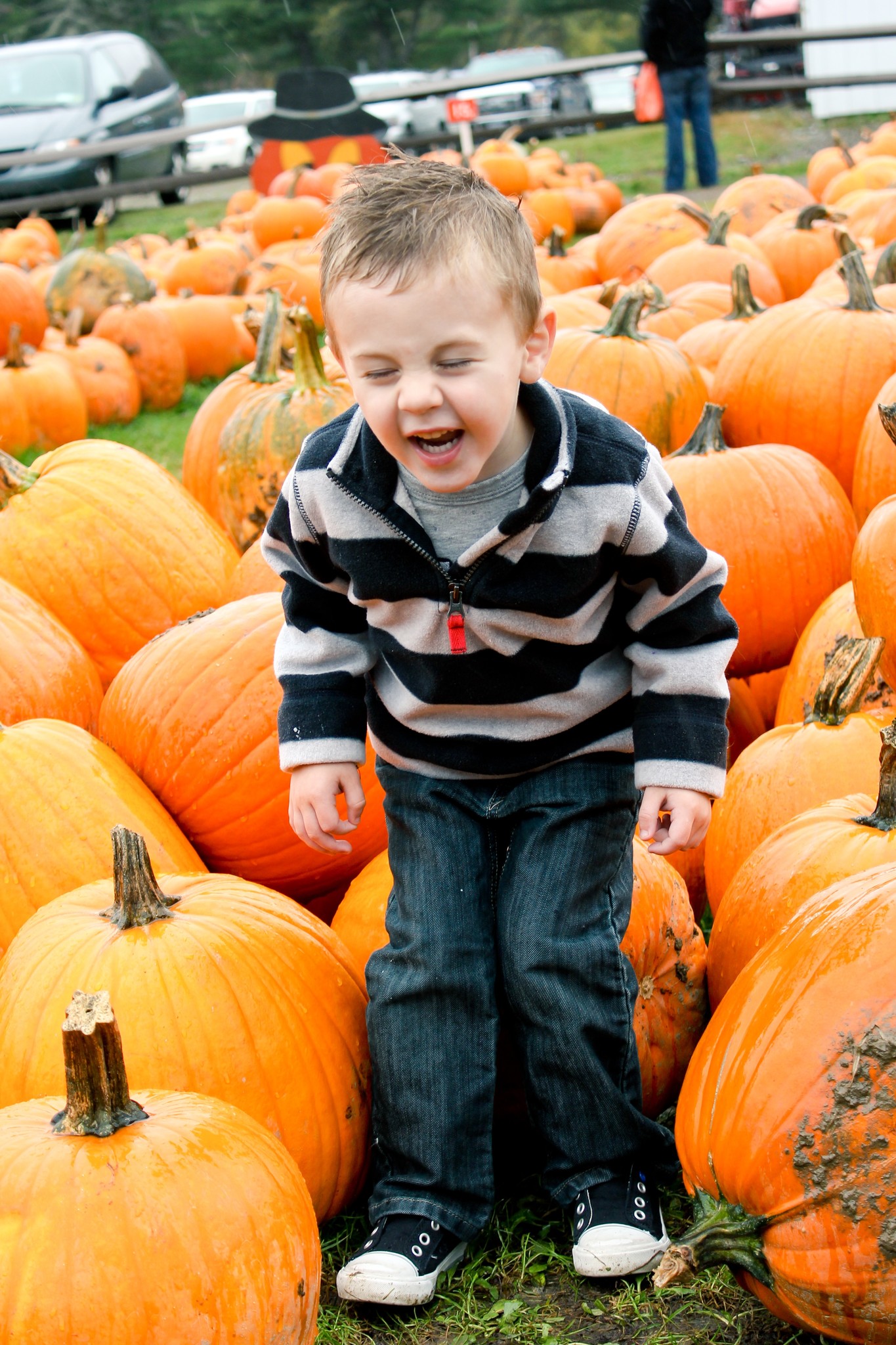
[631,62,664,122]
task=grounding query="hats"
[246,71,388,141]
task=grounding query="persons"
[638,0,719,193]
[262,141,745,1312]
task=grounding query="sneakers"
[563,1162,671,1280]
[335,1213,468,1307]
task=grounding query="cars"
[178,50,668,174]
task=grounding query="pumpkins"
[0,122,895,1345]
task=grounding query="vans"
[0,27,187,226]
